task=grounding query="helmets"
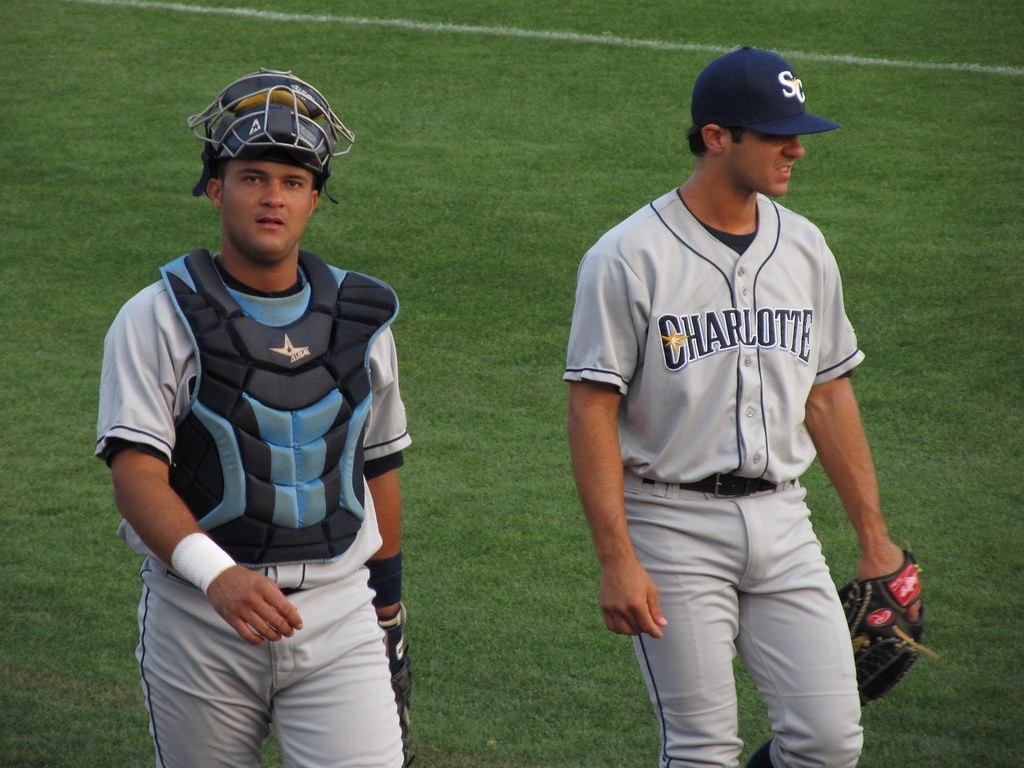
[206,69,338,168]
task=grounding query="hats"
[692,46,840,136]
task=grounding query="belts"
[643,472,795,498]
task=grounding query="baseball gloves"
[837,549,924,705]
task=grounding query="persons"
[563,46,923,768]
[93,68,416,768]
